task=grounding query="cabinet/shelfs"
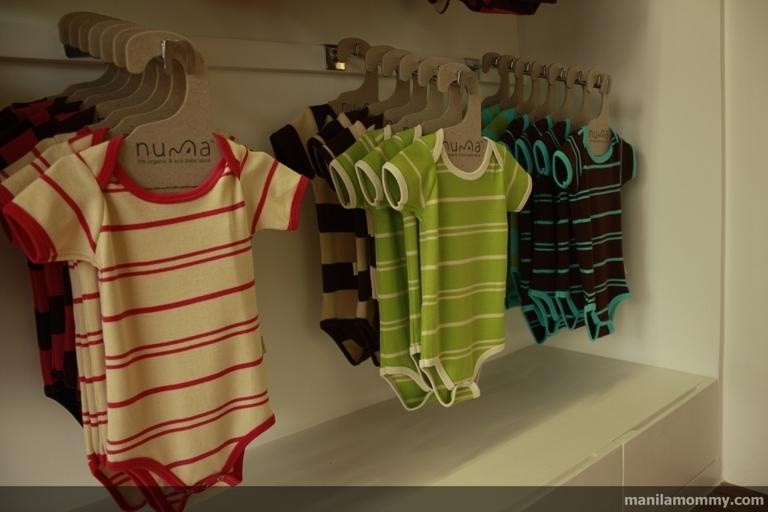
[65,346,722,511]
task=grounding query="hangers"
[477,46,615,158]
[328,38,489,171]
[57,11,231,198]
